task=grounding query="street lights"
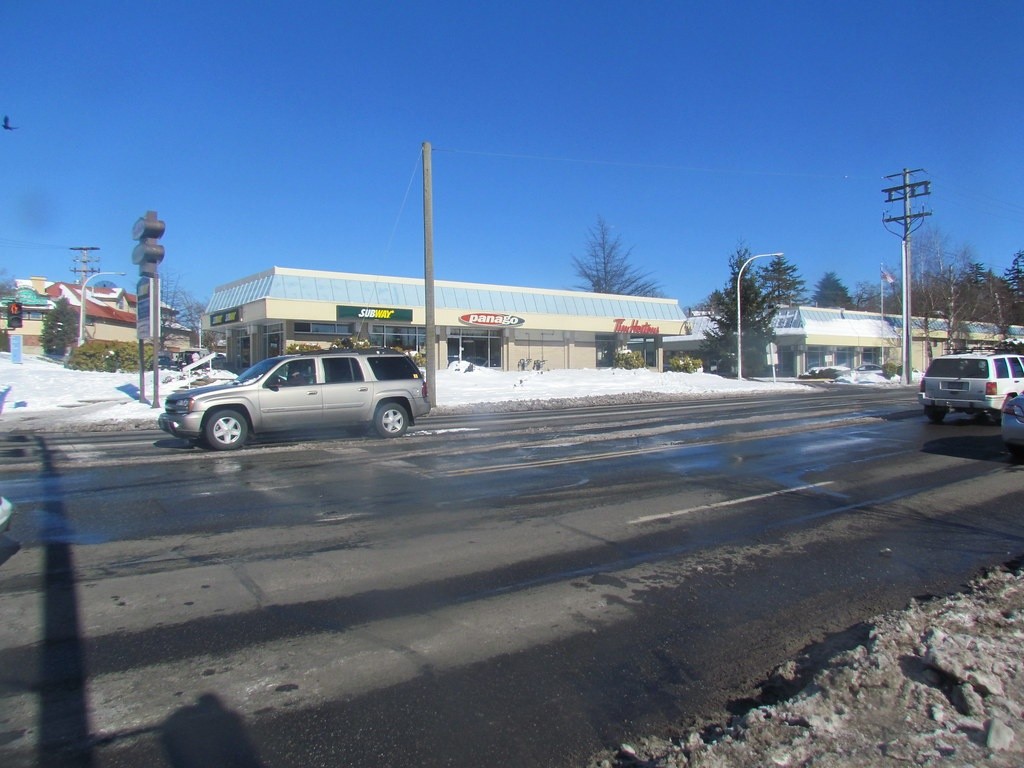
[736,253,784,380]
[78,272,126,348]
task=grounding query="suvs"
[918,347,1023,426]
[157,347,432,453]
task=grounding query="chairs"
[962,359,985,376]
[303,366,314,384]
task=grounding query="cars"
[849,364,885,374]
[911,368,924,381]
[1001,390,1024,455]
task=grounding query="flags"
[880,268,897,286]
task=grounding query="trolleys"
[517,359,548,372]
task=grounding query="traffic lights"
[7,302,24,329]
